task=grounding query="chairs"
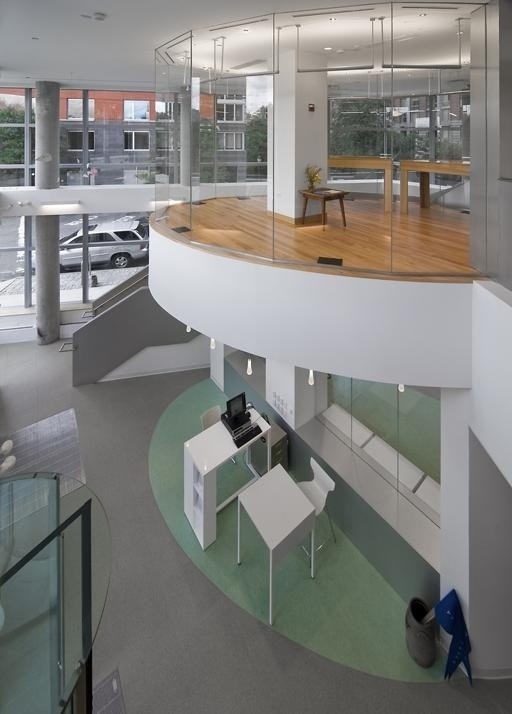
[294,457,336,569]
[200,406,222,430]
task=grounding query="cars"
[31,221,149,272]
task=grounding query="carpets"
[0,408,87,531]
[146,378,470,684]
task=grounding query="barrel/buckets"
[404,596,436,669]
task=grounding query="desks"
[400,159,470,215]
[236,463,313,626]
[182,409,271,551]
[299,188,349,230]
[328,155,393,213]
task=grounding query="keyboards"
[233,424,262,448]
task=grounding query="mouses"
[260,436,266,443]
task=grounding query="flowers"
[304,164,321,184]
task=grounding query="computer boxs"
[221,411,251,437]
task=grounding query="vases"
[308,184,315,192]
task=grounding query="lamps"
[186,325,405,392]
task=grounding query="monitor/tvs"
[226,392,246,419]
